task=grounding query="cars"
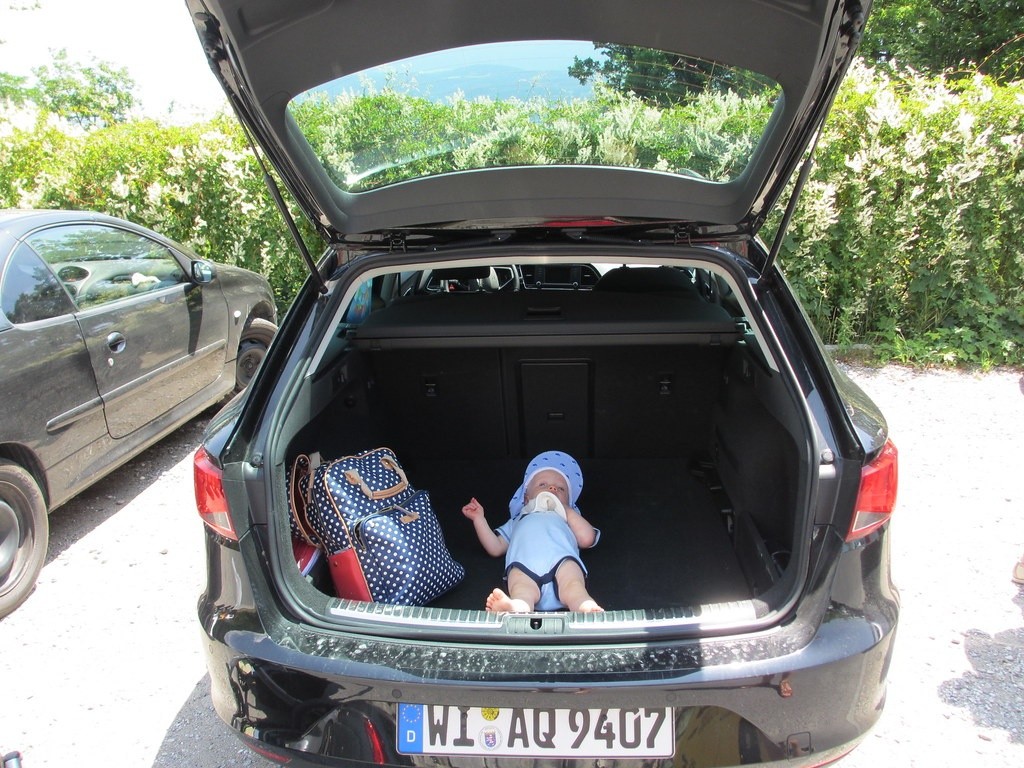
[185,0,903,768]
[0,209,279,621]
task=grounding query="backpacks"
[283,445,465,609]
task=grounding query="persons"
[461,451,605,612]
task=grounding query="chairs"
[592,262,705,300]
[433,267,493,293]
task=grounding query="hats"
[509,450,584,518]
